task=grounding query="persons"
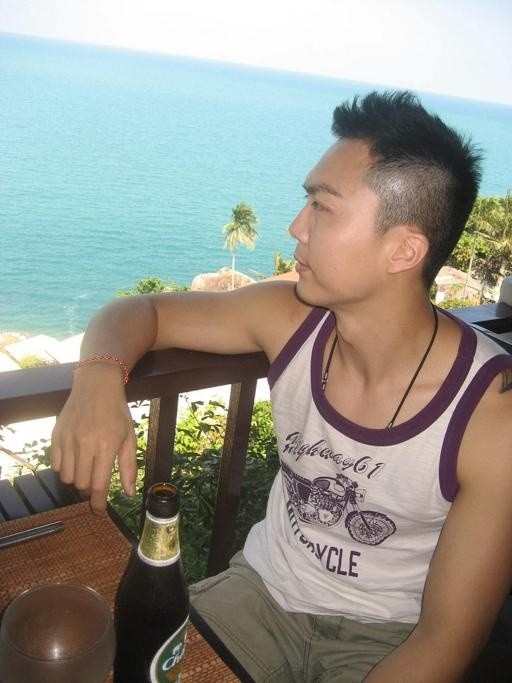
[51,89,510,683]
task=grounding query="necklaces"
[322,302,440,428]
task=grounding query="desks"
[1,467,254,683]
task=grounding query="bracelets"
[73,357,131,387]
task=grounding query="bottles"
[114,482,197,683]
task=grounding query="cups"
[0,581,113,682]
